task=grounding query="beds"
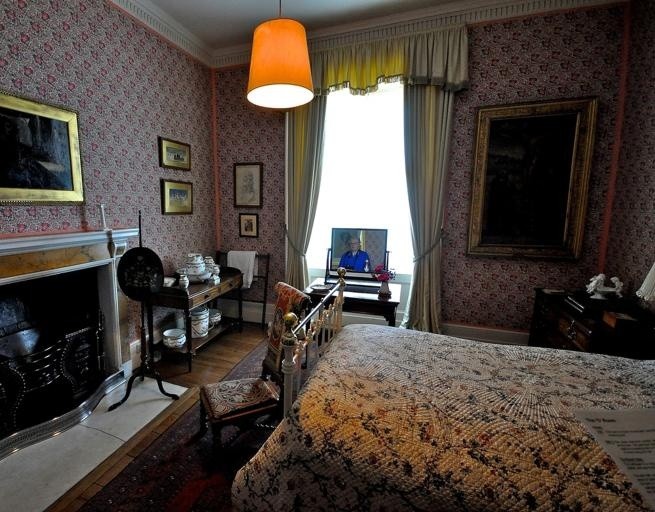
[224,267,654,512]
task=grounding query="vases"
[376,279,392,297]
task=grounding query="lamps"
[240,0,320,109]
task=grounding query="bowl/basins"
[162,276,175,287]
[177,268,212,281]
[164,330,187,349]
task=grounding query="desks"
[145,262,244,372]
[301,272,404,326]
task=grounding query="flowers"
[370,261,398,282]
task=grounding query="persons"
[337,234,373,272]
[586,273,623,298]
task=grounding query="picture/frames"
[237,212,259,239]
[159,177,194,217]
[231,161,265,208]
[0,92,87,207]
[329,224,389,274]
[156,135,192,173]
[464,93,602,261]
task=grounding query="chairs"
[195,279,313,453]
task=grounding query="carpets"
[75,331,325,512]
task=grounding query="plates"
[311,283,333,293]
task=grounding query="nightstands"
[526,278,655,357]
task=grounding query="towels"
[228,249,258,292]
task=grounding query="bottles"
[212,265,219,275]
[180,275,189,289]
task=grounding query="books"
[603,309,638,329]
